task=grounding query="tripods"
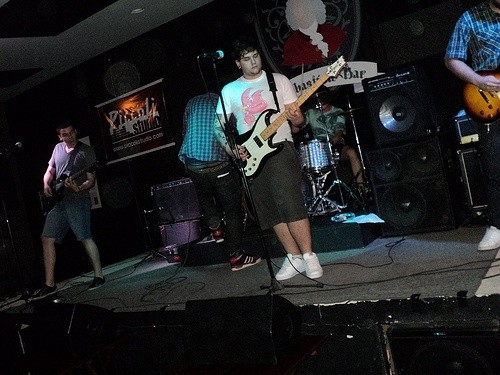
[300,90,365,220]
[210,59,323,291]
[0,197,41,307]
[134,171,167,267]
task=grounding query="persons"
[213,42,324,279]
[178,82,261,272]
[443,0,500,251]
[300,89,366,196]
[30,122,105,301]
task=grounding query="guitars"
[460,64,500,124]
[41,161,99,212]
[227,55,348,178]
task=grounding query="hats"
[316,93,327,108]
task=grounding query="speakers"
[183,292,299,368]
[456,147,489,211]
[150,177,205,223]
[385,326,499,375]
[32,301,116,356]
[345,60,458,238]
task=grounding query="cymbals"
[334,107,361,117]
[308,91,327,102]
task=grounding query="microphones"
[200,50,225,60]
[3,142,22,153]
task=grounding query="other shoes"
[358,186,371,198]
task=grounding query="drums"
[243,171,317,224]
[296,139,336,174]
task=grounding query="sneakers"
[30,284,57,300]
[275,254,306,280]
[302,252,324,278]
[477,226,500,250]
[230,250,261,271]
[87,276,106,290]
[211,228,225,242]
